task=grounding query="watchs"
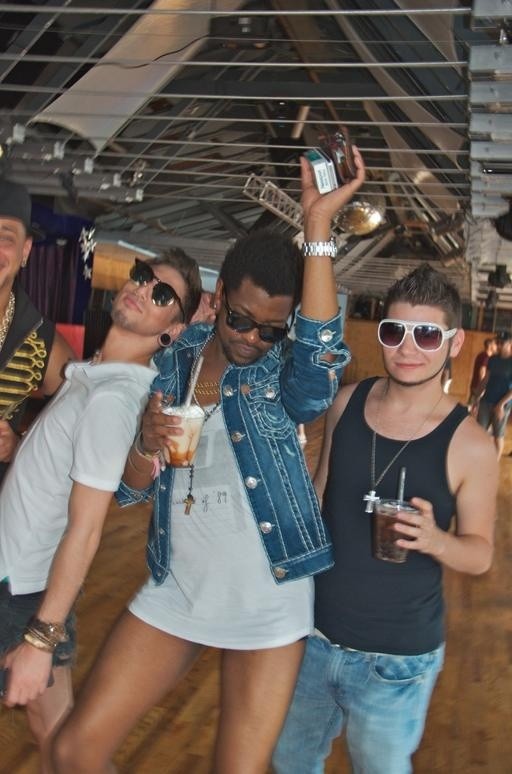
[300,235,341,260]
[28,616,72,644]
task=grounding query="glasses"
[377,319,456,352]
[129,255,187,323]
[223,286,289,343]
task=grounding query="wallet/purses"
[321,126,356,186]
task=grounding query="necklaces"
[362,370,447,514]
[0,291,16,353]
[182,329,223,516]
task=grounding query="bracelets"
[22,626,58,653]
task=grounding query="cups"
[373,499,420,564]
[161,406,206,468]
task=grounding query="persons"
[349,291,368,319]
[48,140,368,774]
[475,328,512,462]
[268,259,500,771]
[0,243,203,771]
[190,292,217,324]
[466,337,497,416]
[0,178,79,491]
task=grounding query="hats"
[494,331,511,340]
[1,186,48,248]
